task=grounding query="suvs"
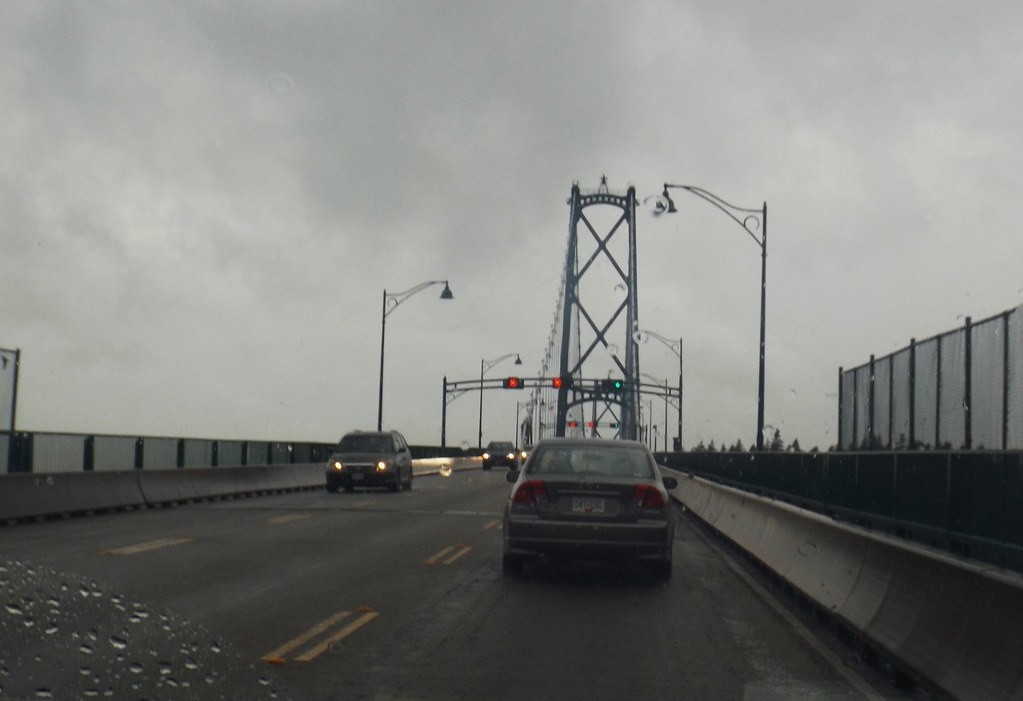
[325,429,413,491]
[482,440,518,469]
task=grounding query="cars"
[502,438,678,572]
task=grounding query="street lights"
[478,353,522,448]
[657,184,768,448]
[378,280,454,431]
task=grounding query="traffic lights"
[610,422,619,428]
[569,422,577,427]
[503,377,525,389]
[611,380,624,391]
[589,421,598,427]
[553,378,562,387]
